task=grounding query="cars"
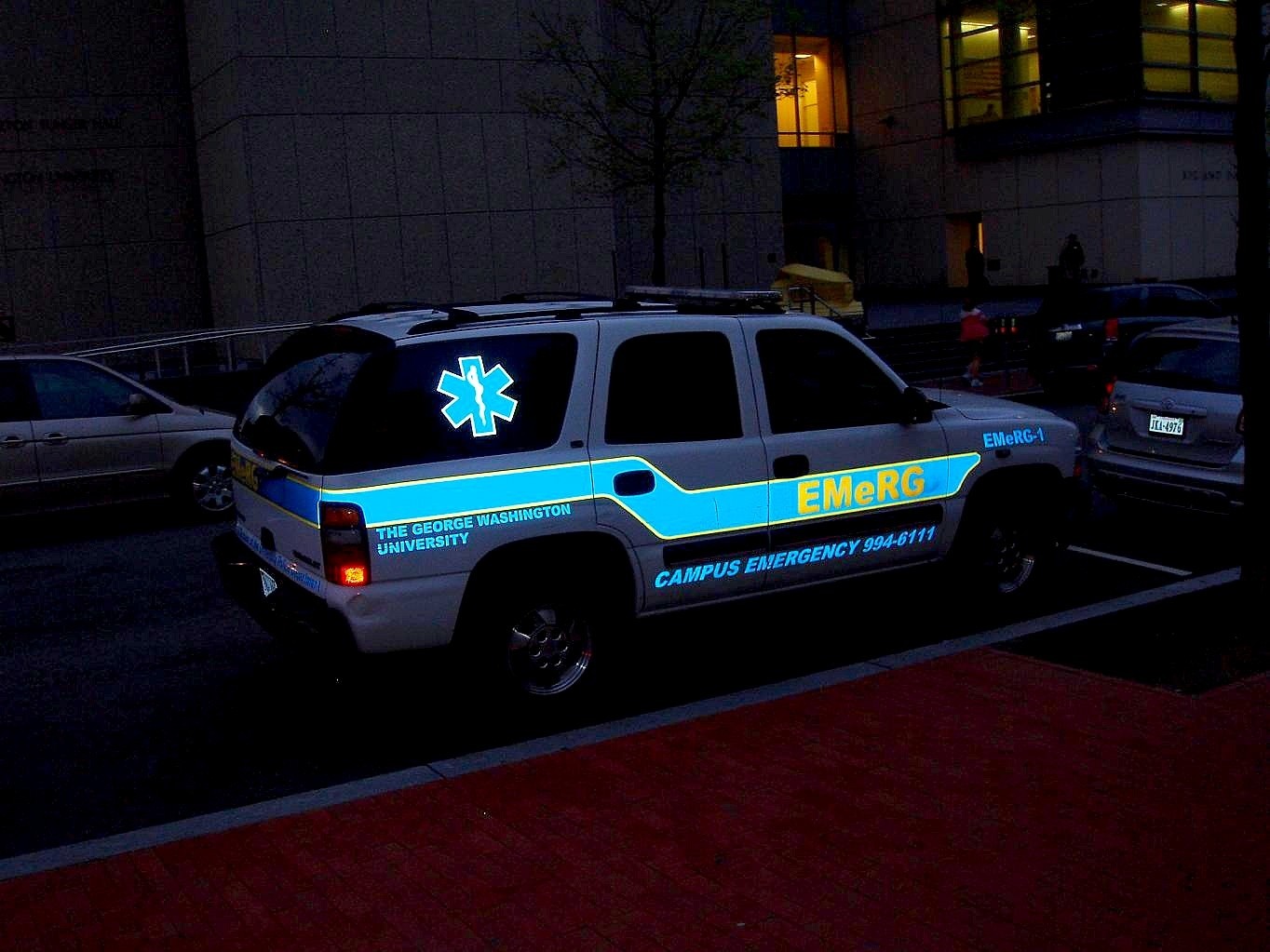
[0,353,237,528]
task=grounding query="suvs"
[1075,314,1244,529]
[1028,281,1225,400]
[215,286,1084,712]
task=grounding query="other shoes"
[971,379,984,387]
[963,372,971,380]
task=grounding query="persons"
[982,104,999,124]
[958,291,991,390]
[1026,239,1086,382]
